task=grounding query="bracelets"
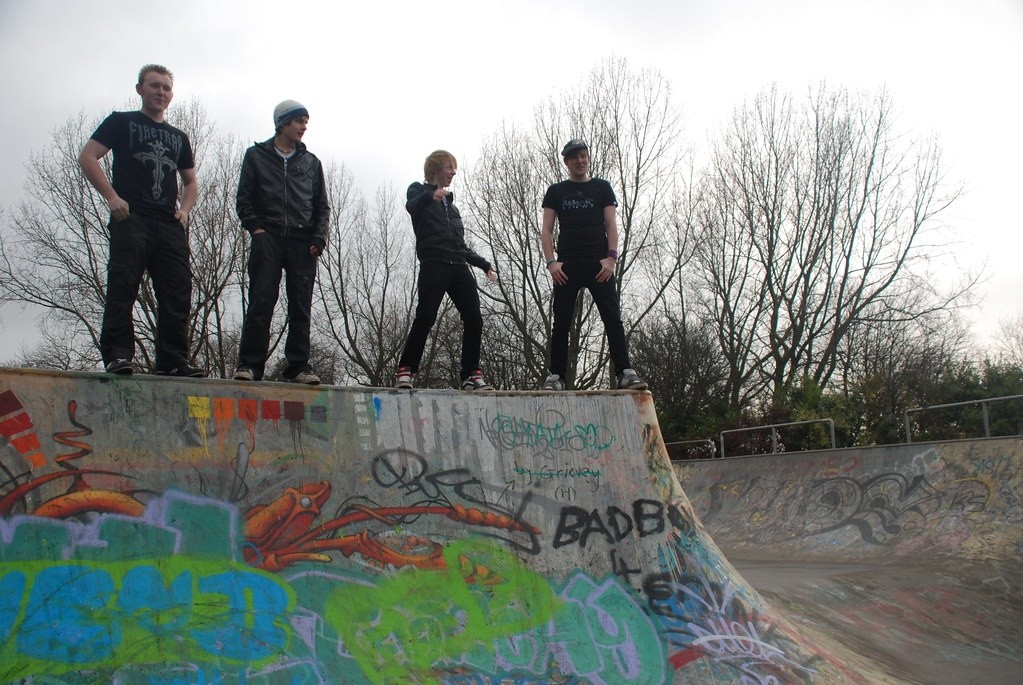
[607,249,618,261]
[545,258,555,269]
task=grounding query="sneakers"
[394,366,416,390]
[105,358,134,374]
[289,369,321,385]
[461,370,494,391]
[152,365,204,378]
[615,369,648,390]
[540,374,566,392]
[231,368,253,381]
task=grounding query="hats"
[561,139,588,156]
[274,99,309,130]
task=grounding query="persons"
[77,64,207,379]
[233,100,331,386]
[391,149,499,392]
[540,139,648,393]
